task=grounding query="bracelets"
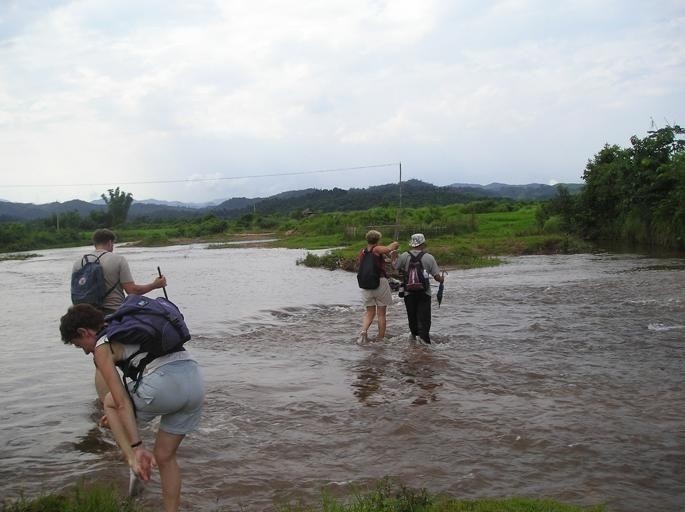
[131,442,143,448]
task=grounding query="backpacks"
[93,293,191,358]
[69,249,120,309]
[404,249,430,292]
[357,245,382,289]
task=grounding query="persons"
[357,229,399,341]
[60,303,204,510]
[389,234,445,345]
[73,228,168,404]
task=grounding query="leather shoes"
[359,330,368,341]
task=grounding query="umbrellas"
[436,270,448,308]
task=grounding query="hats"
[408,233,426,248]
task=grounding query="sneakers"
[128,463,147,498]
